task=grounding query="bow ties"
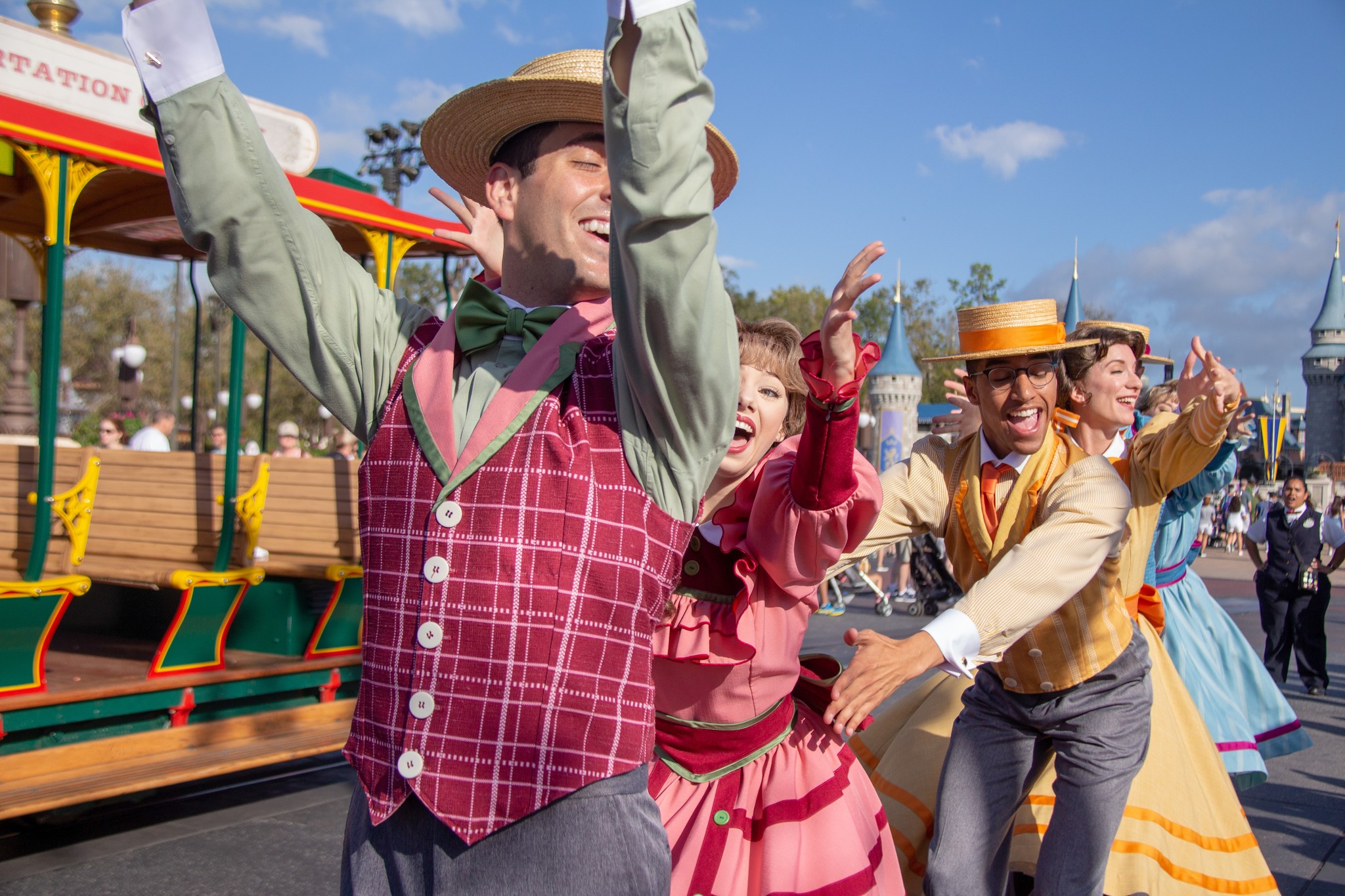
[455,277,570,356]
[1287,510,1301,515]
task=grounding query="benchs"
[47,448,272,680]
[223,457,365,661]
[0,444,102,694]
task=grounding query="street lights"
[355,120,428,211]
[318,405,332,437]
[1261,378,1280,486]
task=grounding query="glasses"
[969,363,1058,391]
[99,428,119,433]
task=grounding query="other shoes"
[876,592,891,604]
[1309,686,1326,695]
[895,593,917,603]
[817,602,845,616]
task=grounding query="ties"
[978,461,1014,542]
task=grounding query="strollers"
[908,535,961,616]
[1208,510,1223,547]
[830,561,892,617]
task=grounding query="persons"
[945,320,1236,587]
[931,535,953,574]
[120,0,739,896]
[129,410,175,452]
[1196,478,1284,557]
[847,326,1282,896]
[327,432,368,461]
[426,185,904,896]
[825,299,1154,896]
[1243,477,1345,694]
[816,539,916,616]
[1324,497,1345,556]
[209,424,227,454]
[99,412,128,449]
[273,420,312,457]
[1128,378,1314,792]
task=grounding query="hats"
[419,49,738,213]
[277,420,299,439]
[1076,320,1176,367]
[922,297,1101,363]
[335,431,357,448]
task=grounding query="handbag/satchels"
[1298,563,1319,593]
